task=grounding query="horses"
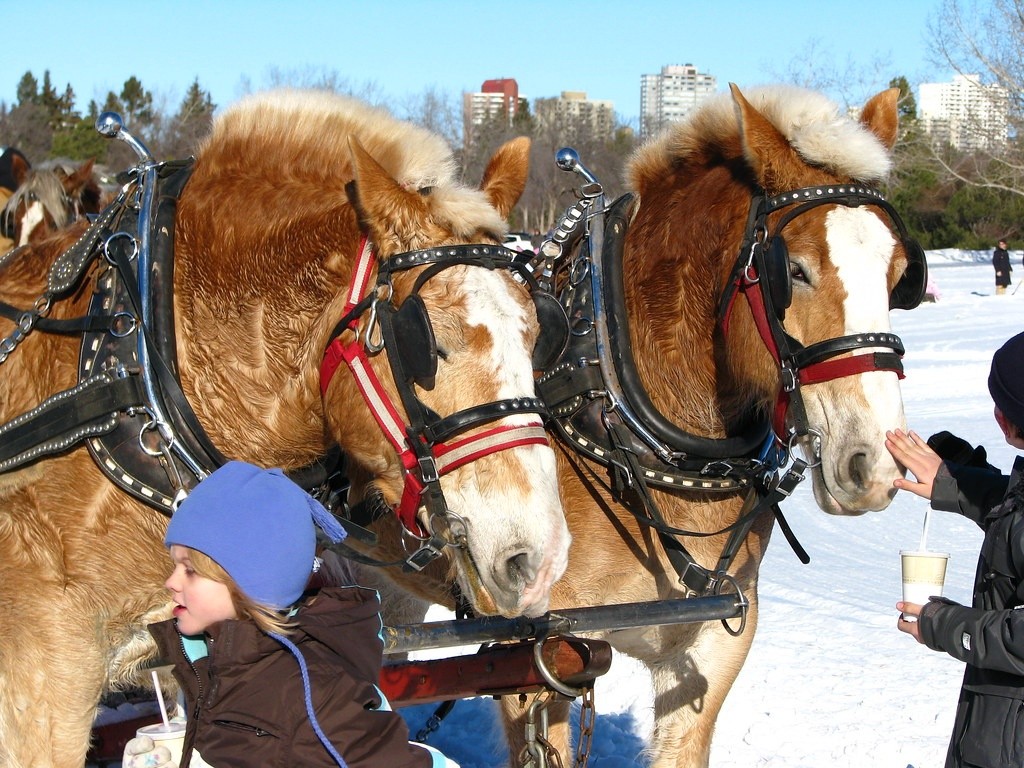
[2,74,930,767]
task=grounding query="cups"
[136,722,186,768]
[900,550,949,622]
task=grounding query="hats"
[164,457,347,611]
[987,329,1024,434]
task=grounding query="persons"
[529,228,545,252]
[884,327,1023,768]
[993,238,1014,296]
[80,459,460,768]
